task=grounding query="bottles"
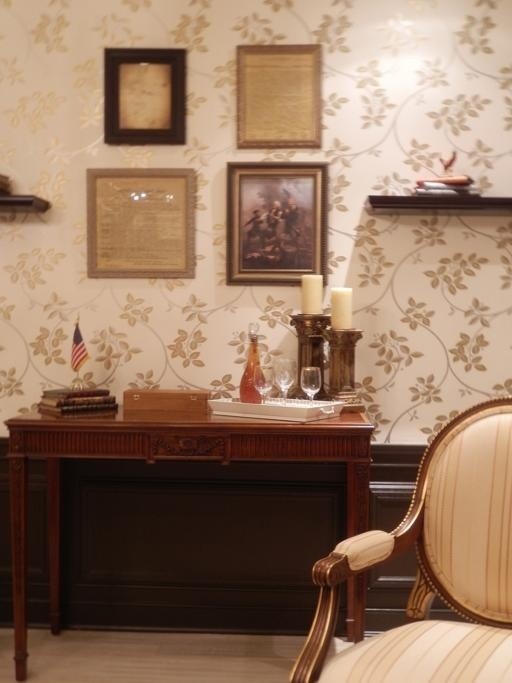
[239,331,266,402]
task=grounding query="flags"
[72,323,90,372]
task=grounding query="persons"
[244,198,298,265]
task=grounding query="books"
[37,387,118,418]
[411,176,480,196]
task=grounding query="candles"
[299,272,325,317]
[328,286,356,331]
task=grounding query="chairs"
[288,394,512,681]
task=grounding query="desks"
[3,401,377,682]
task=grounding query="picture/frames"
[101,43,190,147]
[223,161,328,288]
[84,165,199,281]
[236,42,325,149]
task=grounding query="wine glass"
[253,358,322,406]
